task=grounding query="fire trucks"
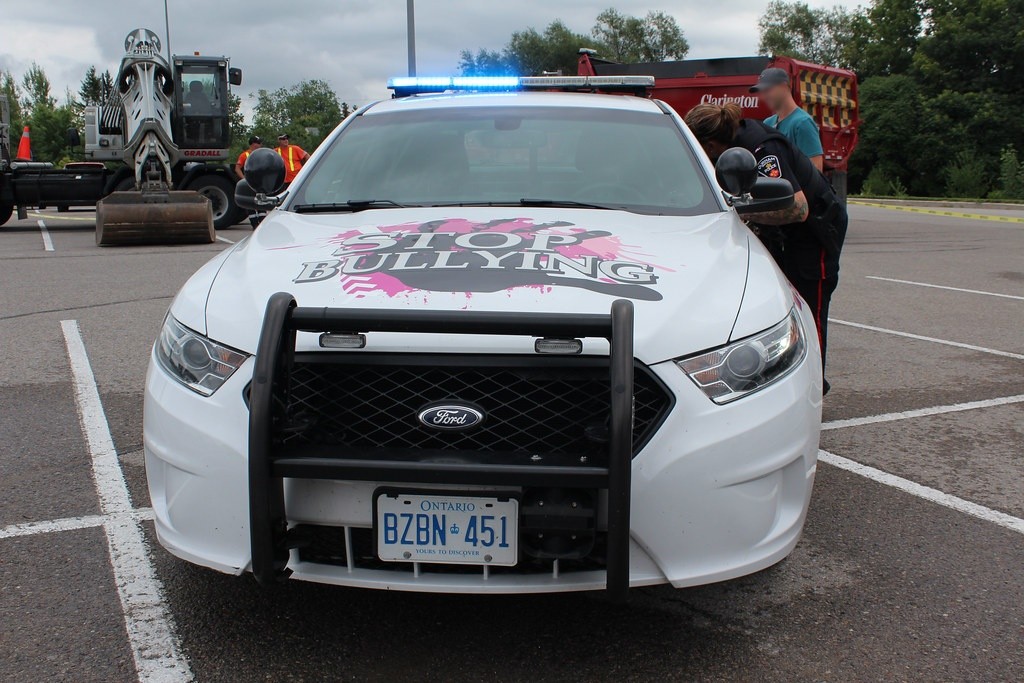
[519,48,858,175]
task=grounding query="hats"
[749,68,789,91]
[249,136,262,145]
[278,134,290,140]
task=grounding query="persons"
[235,134,311,230]
[683,68,849,397]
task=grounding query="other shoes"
[823,379,830,396]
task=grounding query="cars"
[142,75,823,599]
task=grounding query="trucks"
[0,95,252,230]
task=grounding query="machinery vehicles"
[85,25,249,248]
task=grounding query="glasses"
[255,142,260,144]
[279,138,288,140]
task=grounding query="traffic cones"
[15,125,33,161]
